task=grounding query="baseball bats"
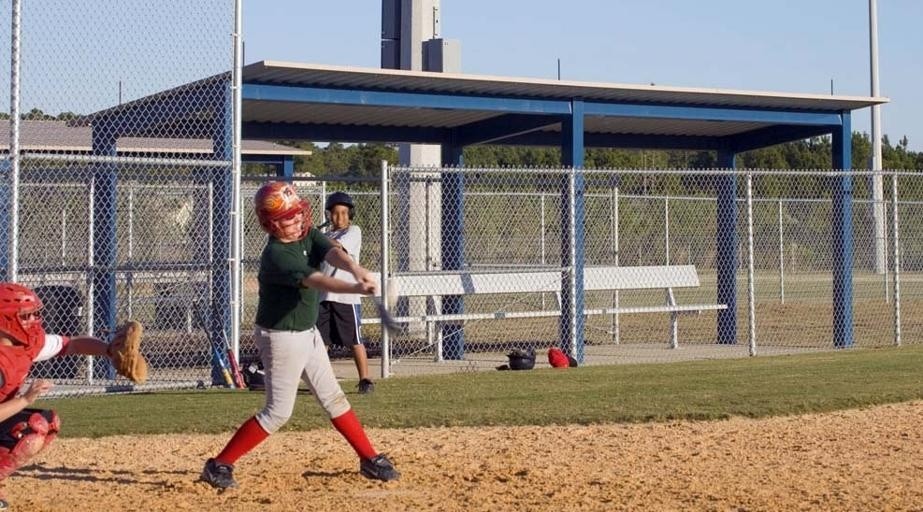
[370,285,403,338]
[193,299,237,389]
[213,299,244,390]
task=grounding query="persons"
[316,190,377,394]
[200,182,400,489]
[1,281,149,512]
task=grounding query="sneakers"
[358,378,375,395]
[200,457,239,491]
[359,454,402,483]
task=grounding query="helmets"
[506,354,536,371]
[326,191,353,210]
[254,182,309,224]
[0,282,44,347]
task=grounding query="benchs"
[360,264,730,362]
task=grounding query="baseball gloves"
[110,320,147,385]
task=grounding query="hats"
[548,348,578,368]
